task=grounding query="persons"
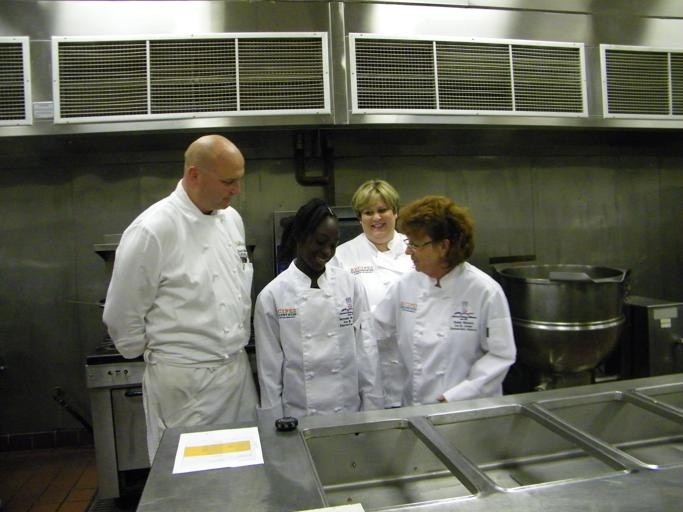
[254,199,385,421]
[372,195,516,408]
[328,179,416,310]
[102,135,260,466]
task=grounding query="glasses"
[403,239,432,249]
